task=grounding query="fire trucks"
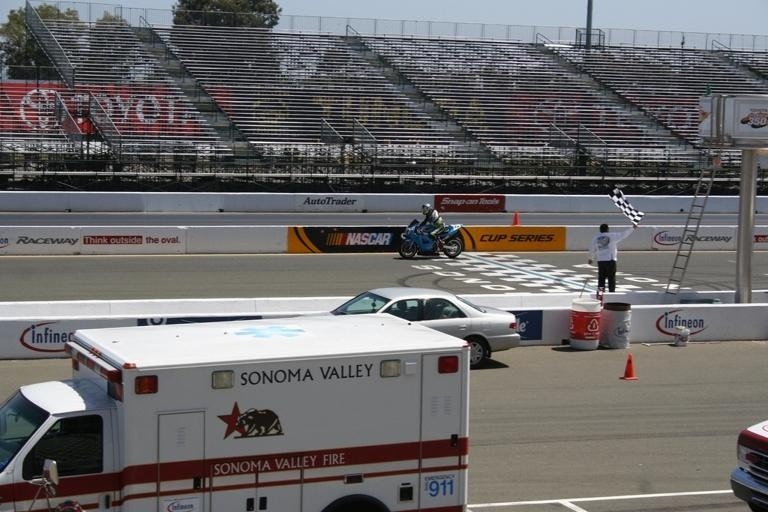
[0,316,477,512]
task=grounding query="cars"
[729,418,768,512]
[297,286,522,368]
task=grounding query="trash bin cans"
[600,302,632,349]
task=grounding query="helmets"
[421,203,432,215]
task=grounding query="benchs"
[1,17,766,169]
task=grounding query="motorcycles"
[400,218,467,258]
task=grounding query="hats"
[599,224,610,233]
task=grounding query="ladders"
[666,141,723,296]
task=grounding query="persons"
[588,222,636,293]
[416,202,448,253]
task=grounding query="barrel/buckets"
[568,298,602,351]
[599,301,632,349]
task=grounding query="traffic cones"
[512,210,521,225]
[618,352,640,380]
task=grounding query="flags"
[608,188,644,226]
